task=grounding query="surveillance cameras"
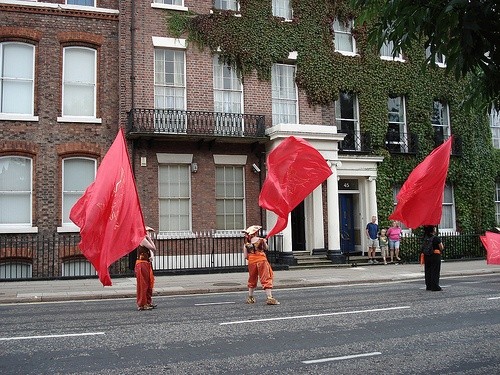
[252,164,261,172]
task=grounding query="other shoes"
[266,297,280,305]
[390,259,393,262]
[147,303,157,307]
[138,305,154,310]
[368,260,373,263]
[248,297,255,304]
[396,257,401,260]
[374,260,379,263]
[384,260,387,265]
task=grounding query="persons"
[135,226,158,311]
[241,225,281,305]
[366,216,403,264]
[420,225,444,291]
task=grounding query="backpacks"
[422,234,437,256]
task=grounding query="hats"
[145,227,155,232]
[246,225,262,235]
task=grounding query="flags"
[388,136,453,229]
[69,128,146,287]
[258,135,334,238]
